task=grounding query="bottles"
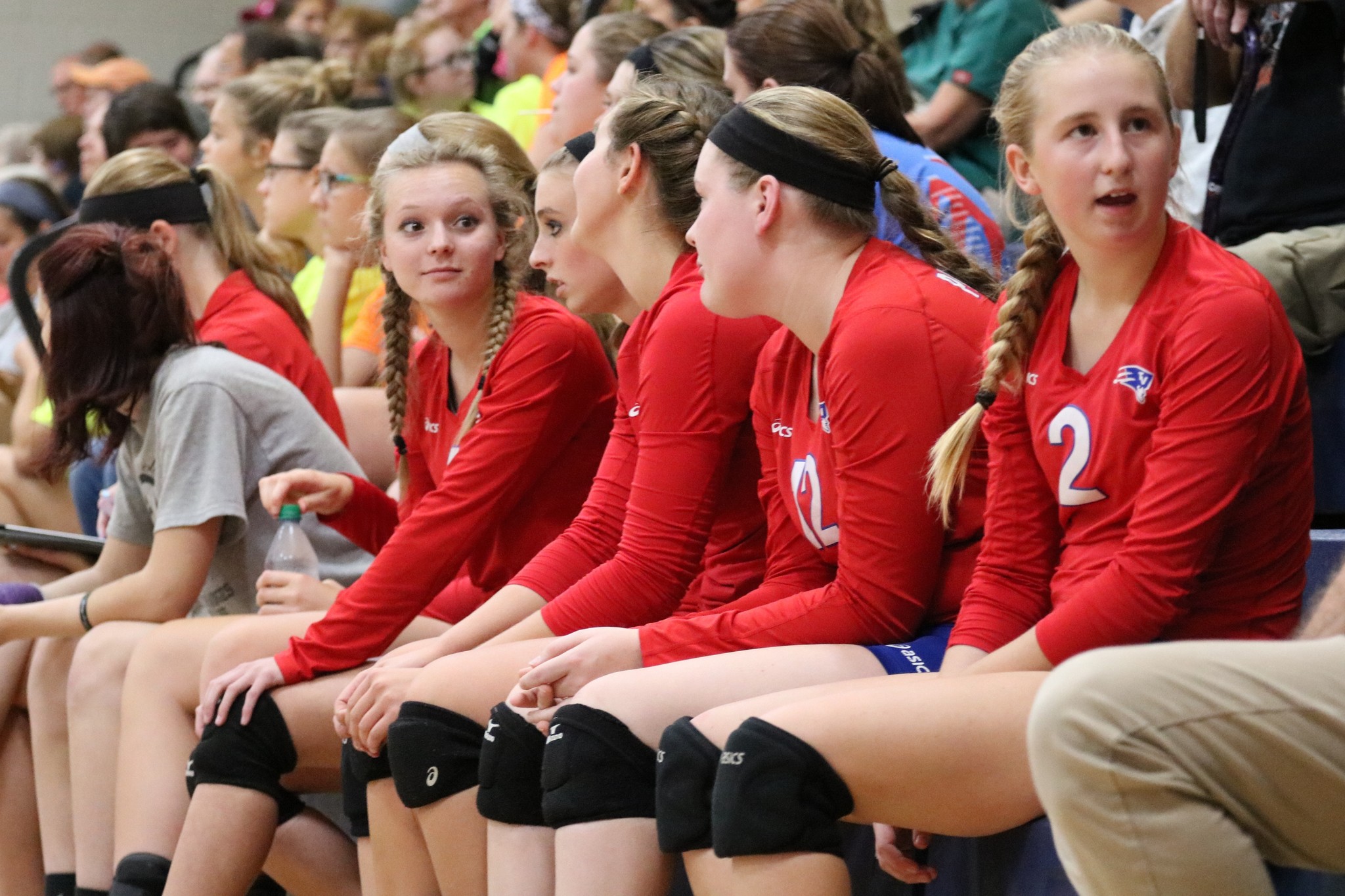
[264,503,320,583]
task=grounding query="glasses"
[264,161,311,177]
[409,49,479,76]
[311,164,373,192]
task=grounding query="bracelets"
[79,590,93,630]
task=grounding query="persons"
[0,223,374,896]
[160,109,614,896]
[476,87,1006,896]
[67,466,486,896]
[0,0,1345,587]
[654,21,1314,896]
[332,132,644,896]
[0,146,348,896]
[1027,559,1345,896]
